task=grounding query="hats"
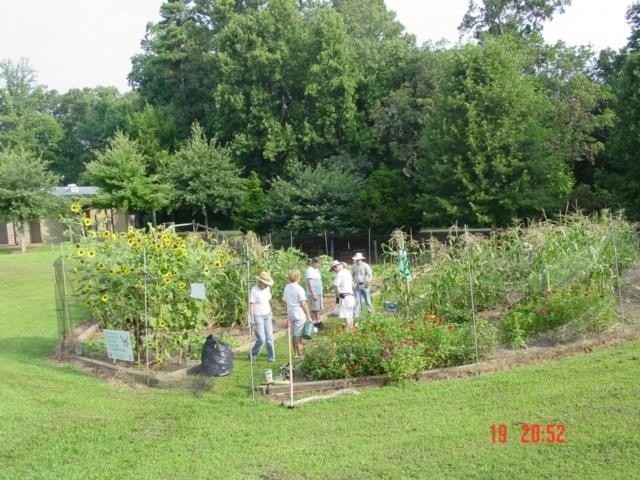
[255,272,273,286]
[328,260,344,272]
[352,252,365,260]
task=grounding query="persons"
[248,252,373,362]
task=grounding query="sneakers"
[312,320,323,330]
[248,356,275,362]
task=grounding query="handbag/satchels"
[339,293,345,298]
[336,298,339,303]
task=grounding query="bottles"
[264,369,272,382]
